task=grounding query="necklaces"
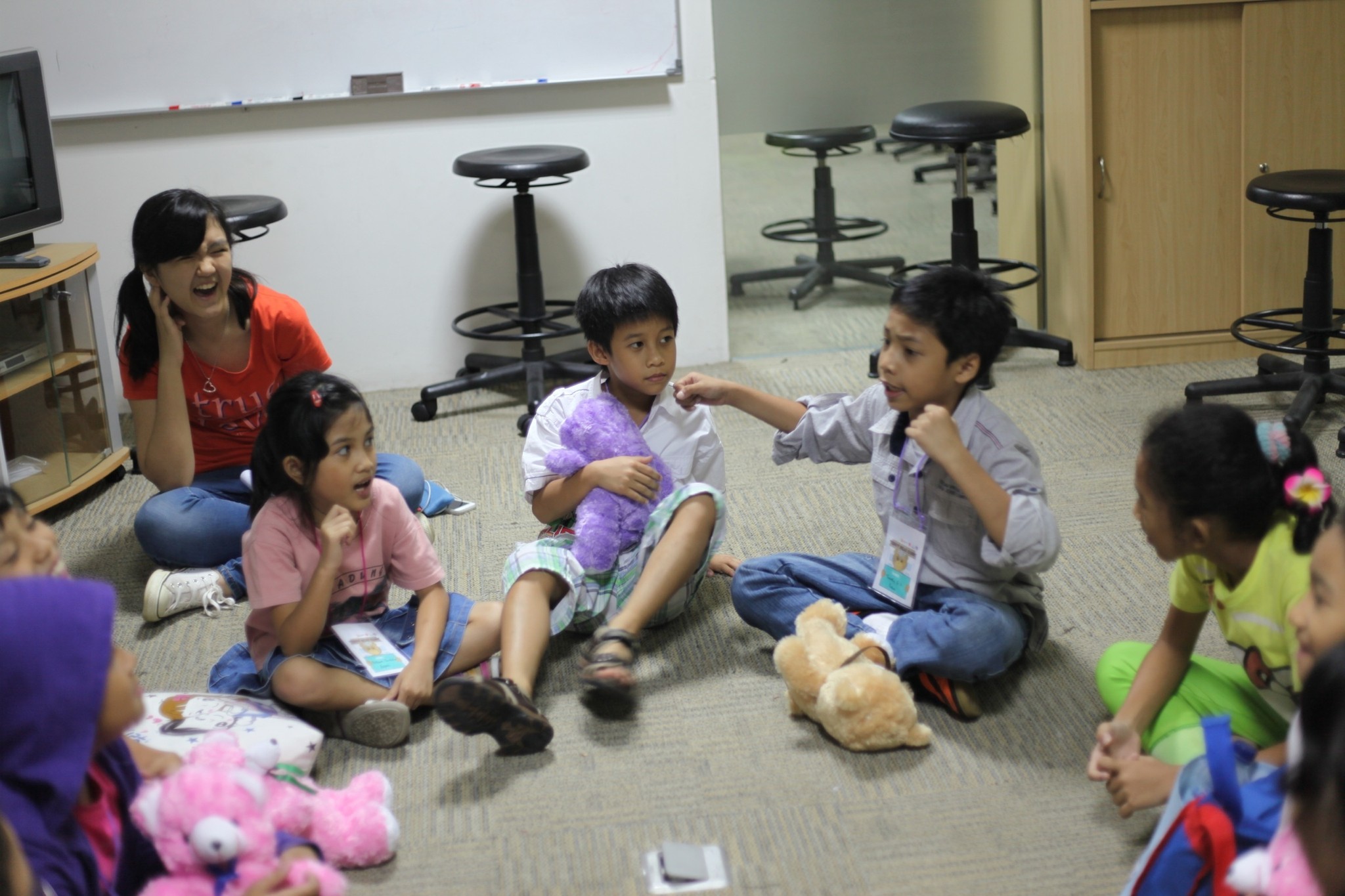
[183,295,228,392]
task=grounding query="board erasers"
[353,72,402,94]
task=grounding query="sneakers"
[903,667,984,718]
[142,566,219,622]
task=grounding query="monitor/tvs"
[0,46,64,256]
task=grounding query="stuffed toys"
[124,732,352,896]
[771,597,933,751]
[542,392,674,565]
[1224,816,1321,896]
[187,731,402,870]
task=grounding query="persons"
[0,482,317,896]
[1282,506,1345,896]
[116,190,438,619]
[432,261,739,756]
[669,259,1063,718]
[1073,399,1339,814]
[244,368,505,749]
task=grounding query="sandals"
[576,627,641,717]
[430,675,555,752]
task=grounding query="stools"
[208,195,288,244]
[866,100,1079,393]
[1178,169,1345,461]
[728,122,908,312]
[410,143,603,438]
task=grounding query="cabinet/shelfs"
[1,240,130,521]
[1040,0,1344,372]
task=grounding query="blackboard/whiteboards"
[0,1,684,120]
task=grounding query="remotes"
[0,255,51,268]
